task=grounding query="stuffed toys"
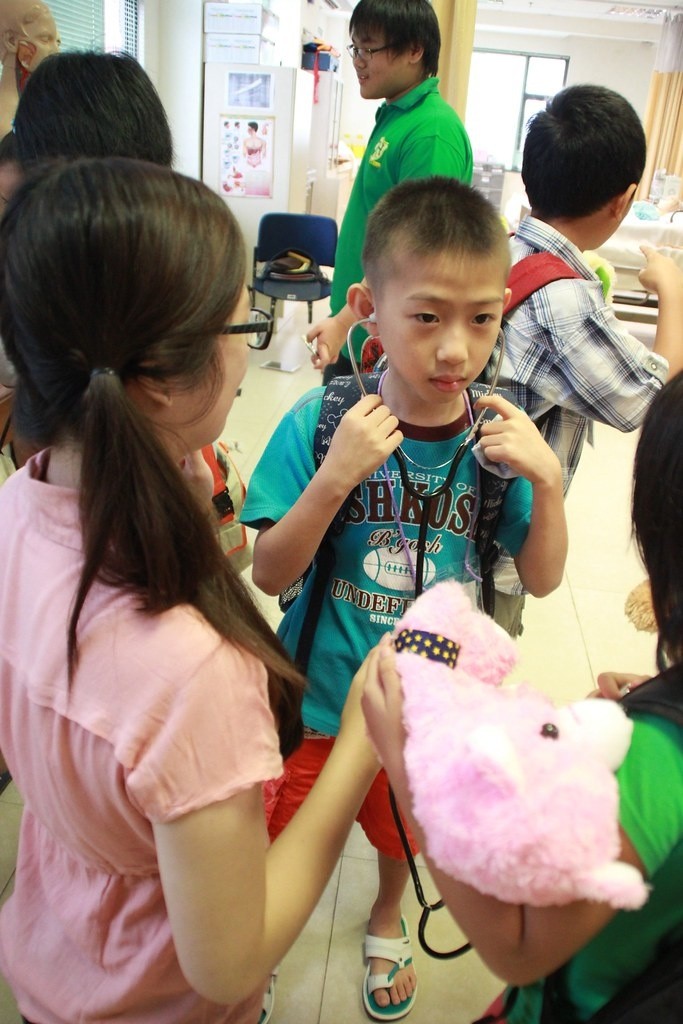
[391,579,647,910]
[582,251,616,308]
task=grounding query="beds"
[518,206,683,311]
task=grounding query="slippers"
[359,914,418,1020]
[255,965,282,1024]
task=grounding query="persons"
[0,0,62,142]
[0,0,683,1024]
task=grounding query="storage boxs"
[304,52,339,72]
[203,1,291,66]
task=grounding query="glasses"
[218,308,277,350]
[346,43,389,61]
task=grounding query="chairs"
[248,211,340,351]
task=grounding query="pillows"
[661,210,683,224]
